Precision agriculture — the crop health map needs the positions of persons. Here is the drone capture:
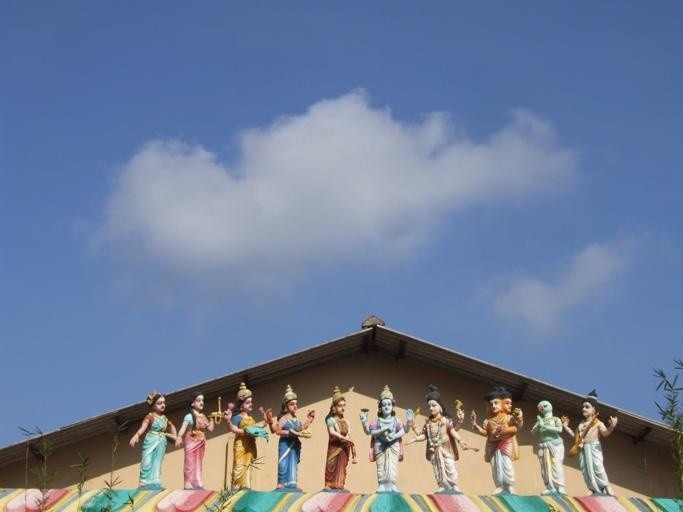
[126,390,176,491]
[359,384,414,493]
[561,388,618,495]
[324,384,357,491]
[266,384,315,493]
[175,390,216,489]
[529,400,567,496]
[470,385,525,496]
[224,381,268,489]
[403,384,481,496]
[500,388,513,413]
